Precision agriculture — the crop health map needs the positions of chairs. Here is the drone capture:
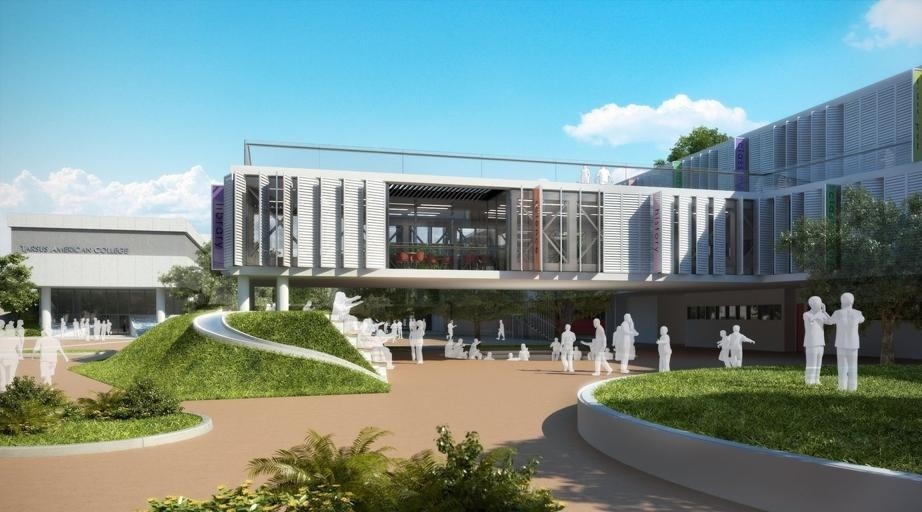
[391,251,488,269]
